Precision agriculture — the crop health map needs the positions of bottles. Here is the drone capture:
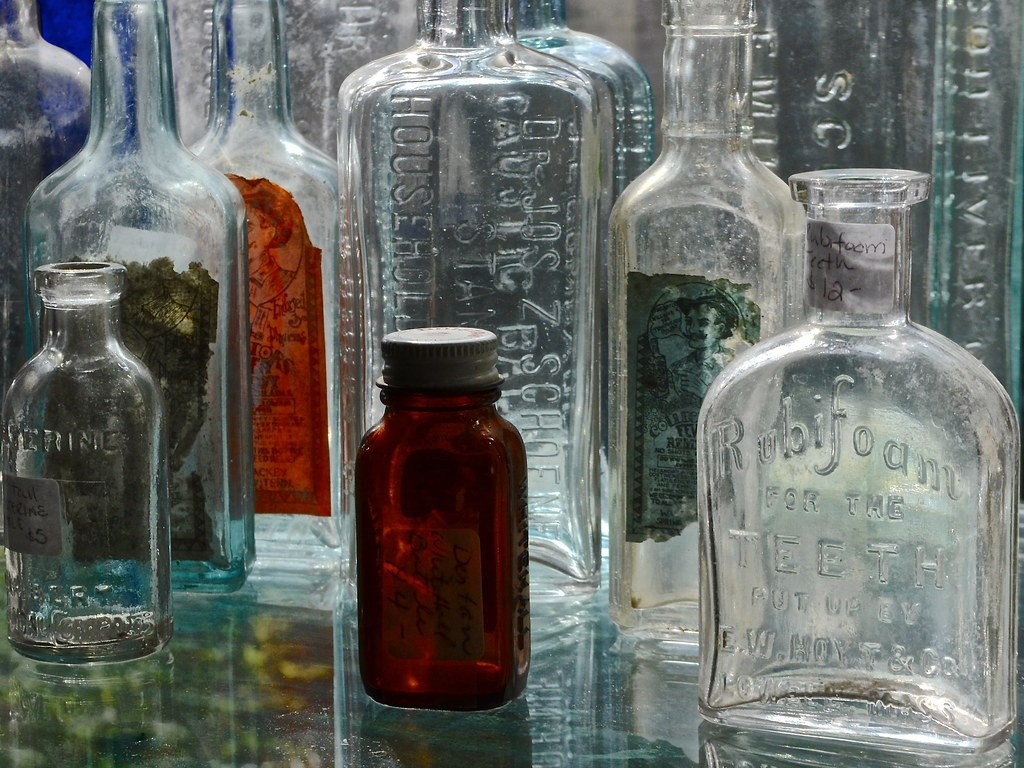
[26,0,254,593]
[608,1,804,645]
[504,0,654,558]
[0,0,92,413]
[189,0,348,573]
[0,259,172,663]
[697,169,1020,753]
[355,326,531,711]
[340,0,601,594]
